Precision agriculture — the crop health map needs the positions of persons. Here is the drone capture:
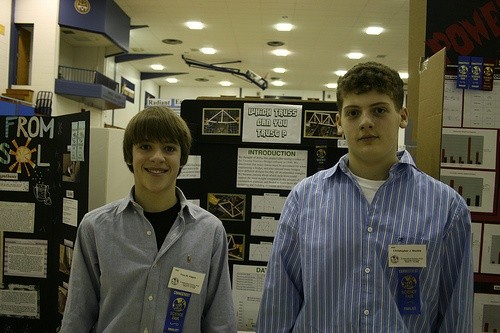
[58,104,238,332]
[253,60,476,333]
